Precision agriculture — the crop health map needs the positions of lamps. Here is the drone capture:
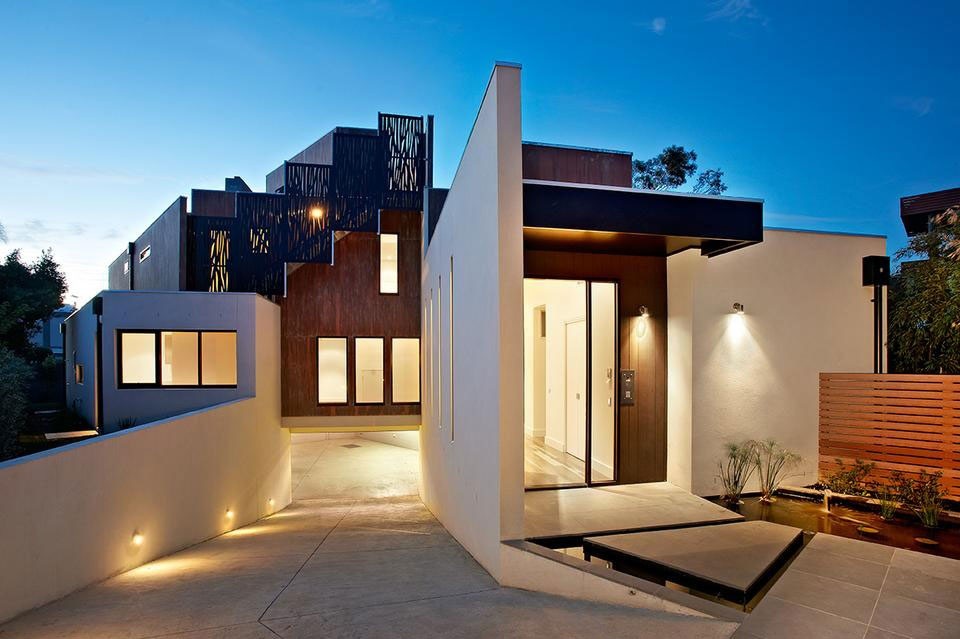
[733,302,744,314]
[639,305,650,317]
